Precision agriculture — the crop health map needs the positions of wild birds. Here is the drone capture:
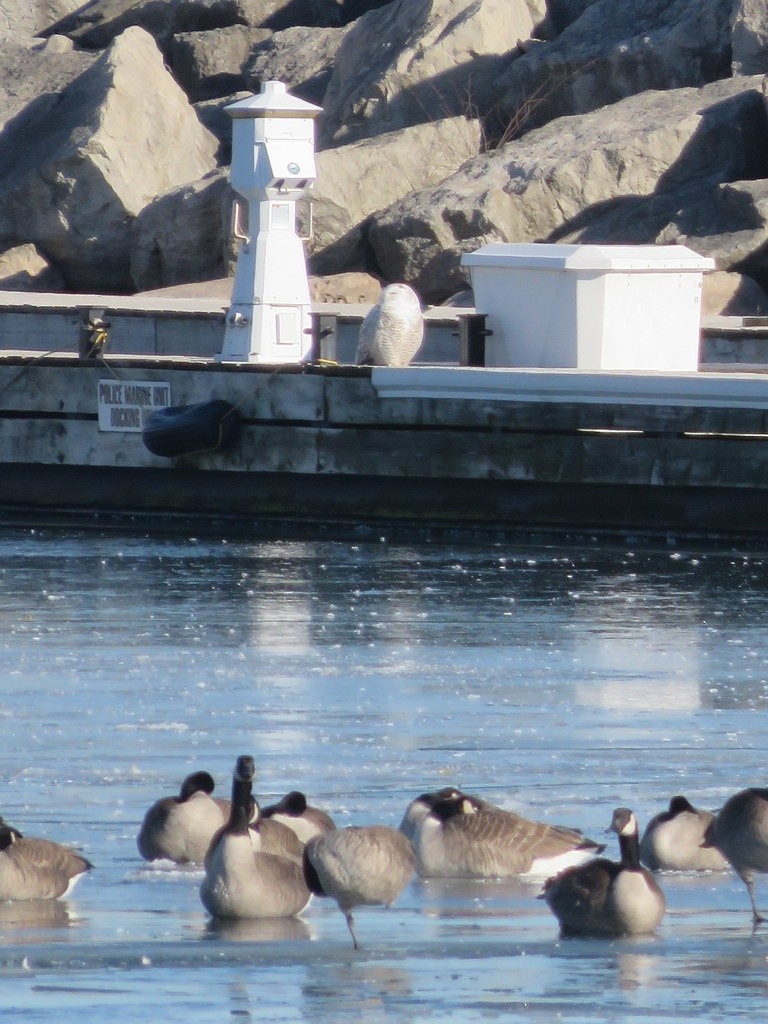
[638,784,768,926]
[0,813,100,902]
[533,806,668,943]
[134,753,609,952]
